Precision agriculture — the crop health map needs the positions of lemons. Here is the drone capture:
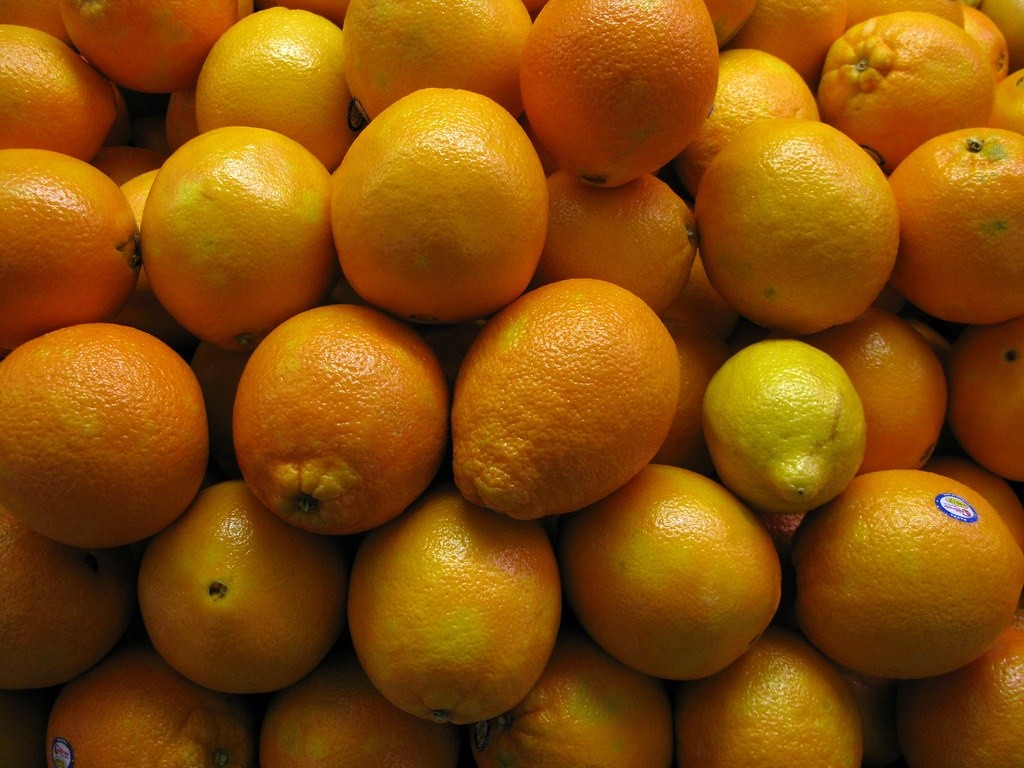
[0,0,1024,768]
